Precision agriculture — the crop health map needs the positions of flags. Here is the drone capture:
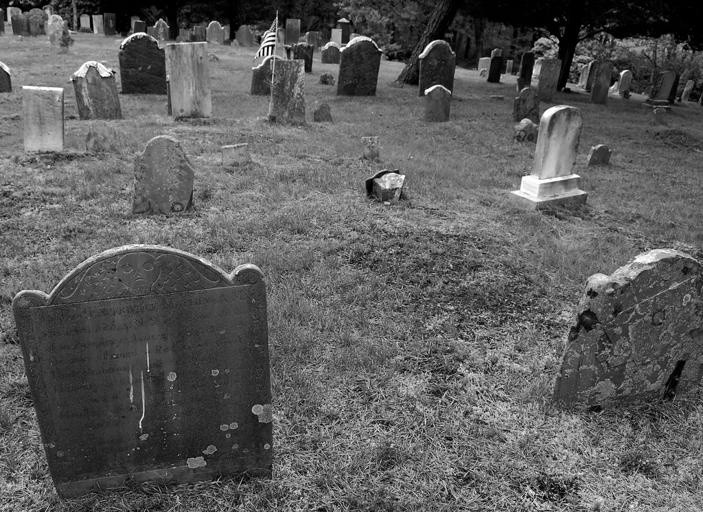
[255,19,276,59]
